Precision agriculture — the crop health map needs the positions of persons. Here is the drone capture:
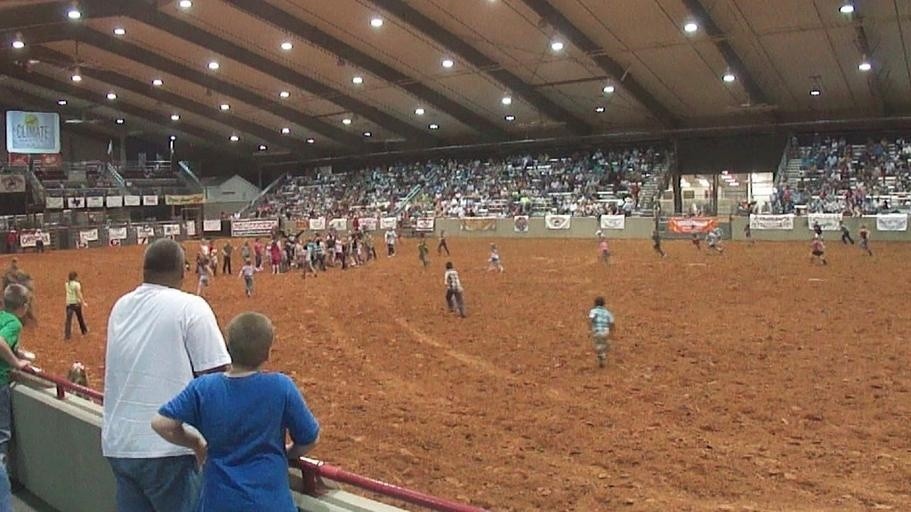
[417,238,431,266]
[150,309,321,512]
[191,225,400,293]
[245,156,446,221]
[769,129,907,267]
[444,262,468,318]
[1,257,34,287]
[587,296,614,366]
[408,145,663,218]
[63,270,89,339]
[647,197,760,258]
[100,237,231,511]
[438,229,449,256]
[36,229,44,252]
[488,242,503,272]
[596,230,609,257]
[1,284,32,492]
